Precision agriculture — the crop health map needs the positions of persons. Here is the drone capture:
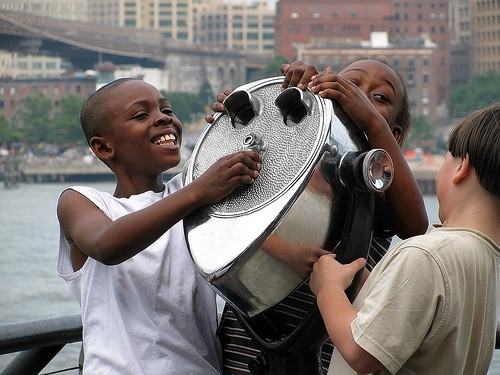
[57,78,262,375]
[218,57,430,375]
[309,100,500,375]
